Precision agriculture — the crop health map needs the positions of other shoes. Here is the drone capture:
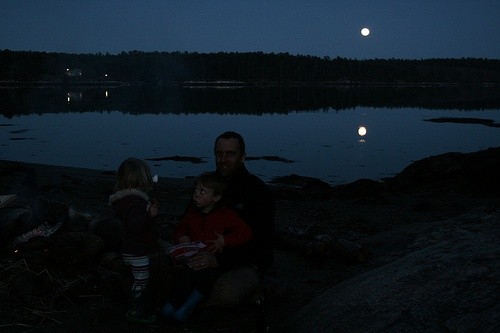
[126,307,157,323]
[174,289,205,321]
[162,303,176,317]
